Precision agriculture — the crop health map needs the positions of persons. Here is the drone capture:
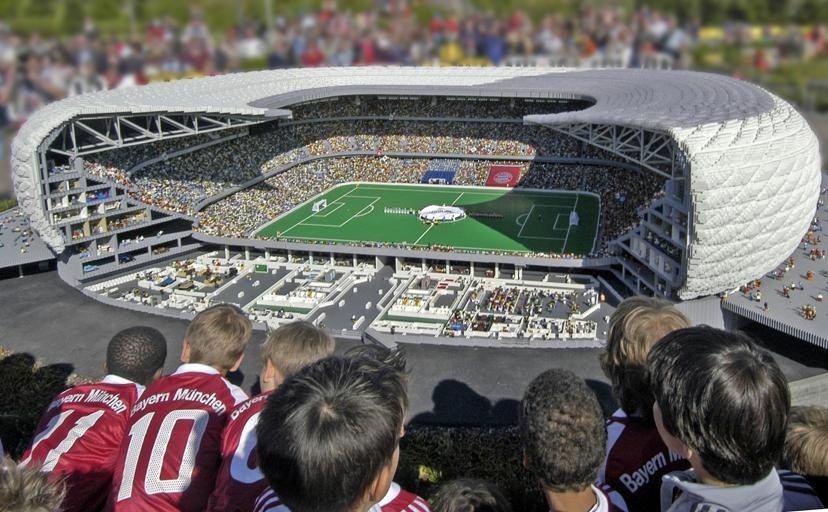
[0,0,827,125]
[737,184,826,320]
[1,299,828,511]
